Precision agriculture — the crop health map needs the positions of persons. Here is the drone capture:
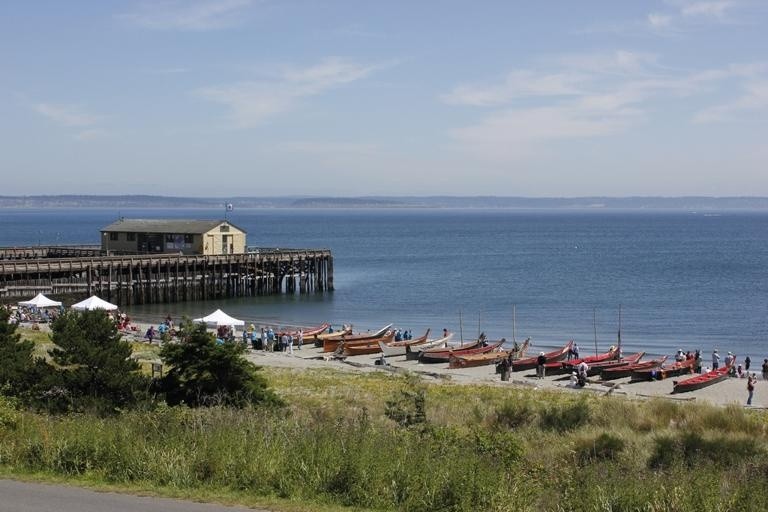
[394,329,412,342]
[6,303,65,323]
[747,375,757,405]
[107,309,173,344]
[501,344,588,388]
[443,328,448,348]
[675,348,768,380]
[215,321,304,353]
[312,334,323,347]
[329,325,333,334]
